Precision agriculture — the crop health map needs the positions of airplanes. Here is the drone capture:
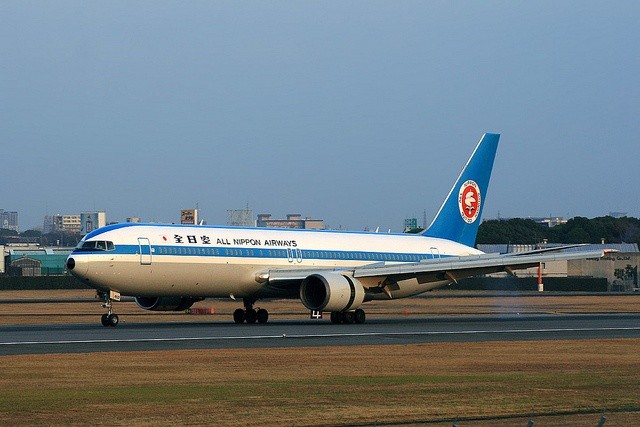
[65,132,619,325]
